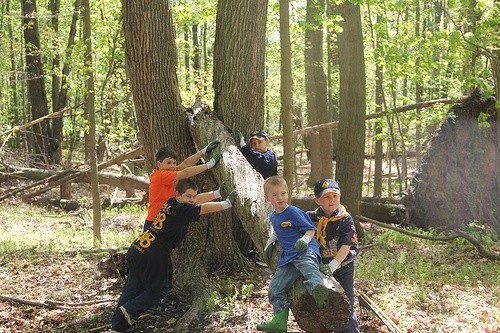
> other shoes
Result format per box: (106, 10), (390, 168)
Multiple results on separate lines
(120, 304), (136, 326)
(111, 327), (138, 333)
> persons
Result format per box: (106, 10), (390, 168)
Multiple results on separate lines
(143, 140), (223, 292)
(256, 176), (329, 333)
(237, 129), (277, 267)
(305, 178), (360, 333)
(110, 178), (238, 333)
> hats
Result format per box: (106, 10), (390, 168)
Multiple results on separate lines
(248, 129), (269, 141)
(314, 178), (341, 199)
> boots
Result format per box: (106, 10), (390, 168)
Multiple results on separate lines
(311, 284), (329, 310)
(256, 309), (289, 332)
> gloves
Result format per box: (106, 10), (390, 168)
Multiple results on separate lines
(205, 150), (222, 170)
(237, 129), (246, 148)
(294, 235), (312, 252)
(263, 234), (278, 264)
(214, 179), (227, 200)
(221, 190), (239, 210)
(201, 139), (220, 156)
(319, 259), (339, 278)
